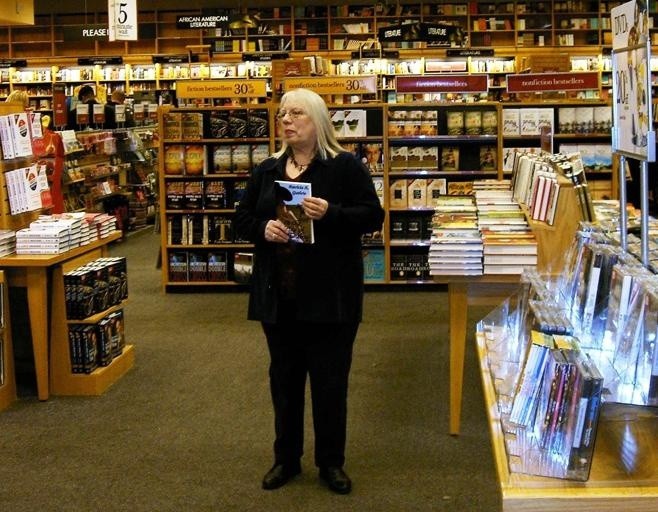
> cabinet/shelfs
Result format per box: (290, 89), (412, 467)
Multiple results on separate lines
(383, 104), (502, 285)
(0, 2), (658, 56)
(499, 104), (615, 197)
(432, 181), (584, 437)
(50, 250), (135, 396)
(161, 54), (658, 102)
(3, 61), (158, 128)
(159, 103), (273, 293)
(273, 103), (386, 286)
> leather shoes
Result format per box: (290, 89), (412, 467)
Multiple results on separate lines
(262, 461), (300, 489)
(319, 467), (353, 493)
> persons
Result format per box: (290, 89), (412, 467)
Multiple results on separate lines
(104, 90), (136, 128)
(228, 86), (386, 494)
(5, 89), (29, 107)
(67, 84), (103, 130)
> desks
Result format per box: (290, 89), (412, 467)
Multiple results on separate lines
(0, 231), (124, 401)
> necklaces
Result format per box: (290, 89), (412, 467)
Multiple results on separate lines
(289, 154), (310, 173)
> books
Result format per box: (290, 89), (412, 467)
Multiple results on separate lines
(257, 23), (290, 34)
(426, 199), (484, 279)
(474, 189), (538, 276)
(51, 130), (77, 152)
(0, 211), (116, 259)
(68, 310), (126, 374)
(507, 266), (604, 479)
(168, 251), (228, 287)
(557, 34), (574, 46)
(330, 108), (368, 137)
(233, 252), (253, 286)
(63, 256), (129, 320)
(4, 166), (54, 215)
(387, 109), (497, 135)
(390, 214), (430, 242)
(390, 254), (432, 281)
(601, 2), (620, 12)
(560, 143), (612, 172)
(168, 180), (246, 209)
(214, 39), (246, 53)
(554, 221), (658, 406)
(165, 109), (268, 138)
(167, 214), (249, 244)
(570, 17), (598, 29)
(517, 19), (526, 30)
(335, 5), (373, 17)
(272, 179), (316, 246)
(361, 247), (385, 280)
(258, 38), (291, 51)
(343, 22), (369, 34)
(163, 144), (269, 174)
(390, 177), (511, 209)
(517, 33), (545, 47)
(388, 145), (498, 170)
(362, 217), (386, 244)
(341, 142), (383, 172)
(0, 113), (44, 159)
(371, 176), (384, 209)
(517, 4), (527, 13)
(554, 0), (585, 12)
(333, 40), (374, 50)
(602, 18), (611, 29)
(503, 105), (613, 134)
(503, 147), (597, 229)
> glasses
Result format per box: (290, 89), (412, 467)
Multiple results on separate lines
(277, 107), (306, 120)
